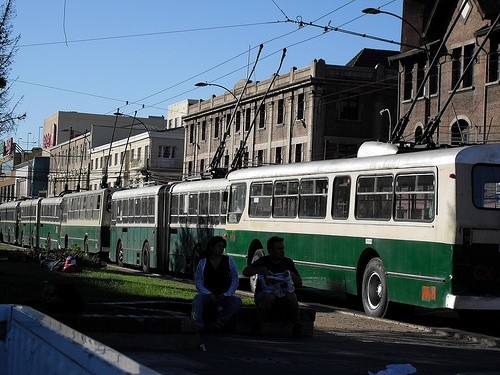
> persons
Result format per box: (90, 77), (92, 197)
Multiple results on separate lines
(242, 236), (307, 338)
(190, 236), (243, 335)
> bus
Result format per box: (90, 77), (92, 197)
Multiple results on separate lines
(1, 1), (500, 321)
